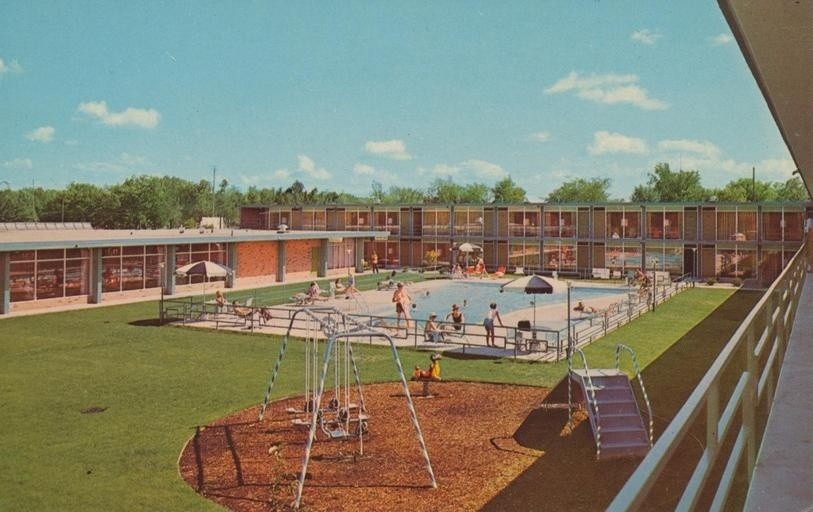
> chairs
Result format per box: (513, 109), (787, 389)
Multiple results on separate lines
(207, 275), (412, 327)
(490, 264), (507, 280)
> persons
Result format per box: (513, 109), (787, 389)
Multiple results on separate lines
(372, 249), (379, 273)
(446, 304), (464, 331)
(452, 254), (484, 274)
(631, 270), (652, 290)
(233, 293), (261, 315)
(803, 207), (813, 273)
(424, 313), (442, 341)
(288, 267), (359, 300)
(392, 281), (412, 339)
(215, 289), (232, 308)
(484, 305), (504, 347)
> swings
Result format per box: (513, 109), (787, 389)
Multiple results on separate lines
(283, 308), (371, 440)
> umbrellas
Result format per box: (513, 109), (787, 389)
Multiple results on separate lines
(175, 260), (232, 303)
(501, 274), (574, 327)
(450, 245), (484, 265)
